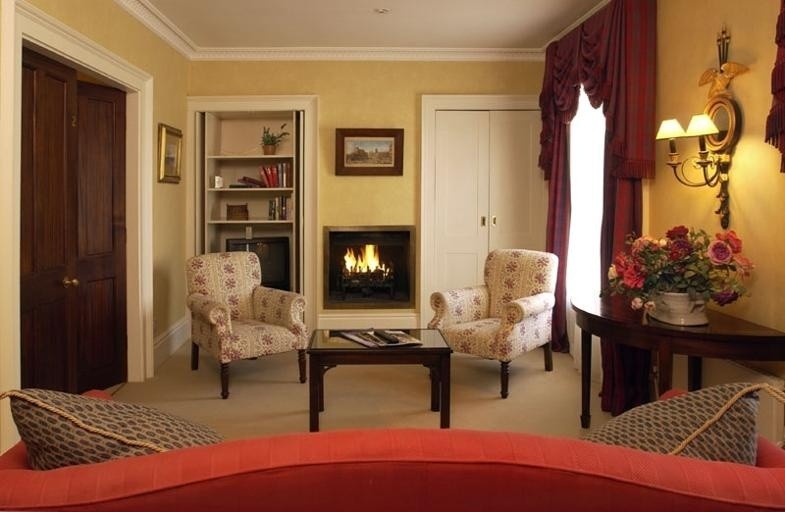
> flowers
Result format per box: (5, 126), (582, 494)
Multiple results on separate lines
(598, 225), (755, 311)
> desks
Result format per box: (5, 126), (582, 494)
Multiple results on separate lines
(570, 294), (785, 429)
(306, 329), (453, 429)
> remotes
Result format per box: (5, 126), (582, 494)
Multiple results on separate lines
(374, 329), (401, 343)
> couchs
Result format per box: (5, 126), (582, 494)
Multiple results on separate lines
(0, 389), (785, 511)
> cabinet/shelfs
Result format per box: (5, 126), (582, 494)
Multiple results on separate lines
(420, 95), (548, 348)
(204, 112), (296, 292)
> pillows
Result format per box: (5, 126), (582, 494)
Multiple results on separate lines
(579, 382), (768, 466)
(0, 388), (228, 471)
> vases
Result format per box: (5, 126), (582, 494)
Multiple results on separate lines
(647, 291), (709, 326)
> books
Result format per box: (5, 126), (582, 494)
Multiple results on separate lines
(229, 161), (293, 221)
(340, 330), (423, 350)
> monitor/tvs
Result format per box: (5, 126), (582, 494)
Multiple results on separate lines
(226, 236), (290, 291)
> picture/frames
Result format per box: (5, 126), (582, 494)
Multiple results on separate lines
(158, 122), (183, 183)
(335, 127), (403, 177)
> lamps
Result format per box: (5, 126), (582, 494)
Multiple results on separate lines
(654, 113), (720, 188)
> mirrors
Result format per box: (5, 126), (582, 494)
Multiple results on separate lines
(701, 94), (738, 153)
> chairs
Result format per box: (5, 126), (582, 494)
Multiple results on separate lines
(426, 249), (559, 398)
(186, 251), (310, 399)
